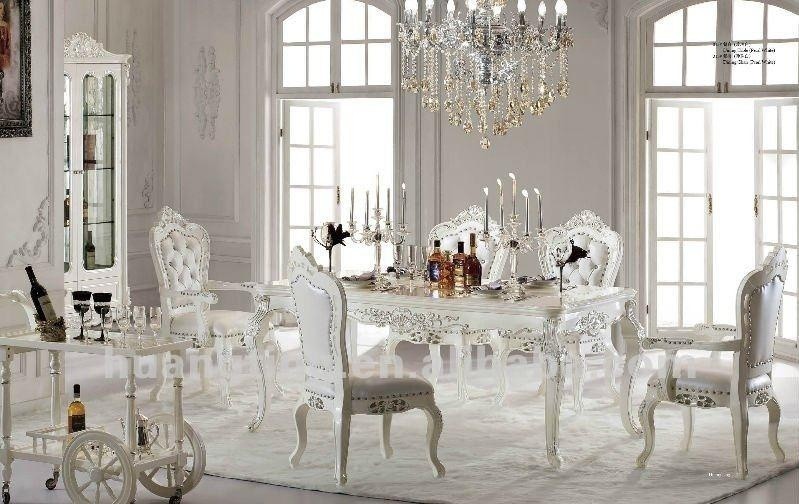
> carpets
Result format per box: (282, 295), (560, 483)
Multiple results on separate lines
(0, 326), (799, 504)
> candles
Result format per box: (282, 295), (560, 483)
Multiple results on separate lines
(509, 173), (516, 215)
(534, 187), (542, 230)
(522, 189), (529, 234)
(376, 172), (379, 209)
(402, 182), (405, 225)
(365, 191), (369, 225)
(483, 185), (489, 231)
(350, 187), (354, 222)
(386, 188), (390, 221)
(497, 178), (503, 228)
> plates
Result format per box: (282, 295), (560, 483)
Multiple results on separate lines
(341, 279), (374, 289)
(522, 281), (558, 291)
(470, 290), (504, 297)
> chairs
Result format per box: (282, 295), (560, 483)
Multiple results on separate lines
(149, 205), (303, 409)
(287, 246), (446, 490)
(411, 205), (510, 402)
(493, 210), (624, 414)
(636, 244), (788, 480)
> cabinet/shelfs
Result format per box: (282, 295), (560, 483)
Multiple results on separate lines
(64, 32), (132, 317)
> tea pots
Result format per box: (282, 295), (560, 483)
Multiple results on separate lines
(118, 408), (159, 460)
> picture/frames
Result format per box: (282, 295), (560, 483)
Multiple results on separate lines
(0, 0), (32, 138)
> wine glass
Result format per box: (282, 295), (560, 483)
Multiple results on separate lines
(79, 306), (92, 346)
(71, 290), (91, 341)
(133, 305), (143, 318)
(149, 305), (161, 339)
(407, 246), (417, 293)
(393, 244), (403, 287)
(94, 293), (112, 343)
(131, 315), (146, 350)
(422, 246), (431, 286)
(115, 306), (131, 349)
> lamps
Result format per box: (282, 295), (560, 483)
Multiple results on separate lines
(396, 0), (576, 150)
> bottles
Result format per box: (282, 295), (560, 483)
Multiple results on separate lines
(83, 191), (88, 223)
(64, 189), (70, 226)
(25, 266), (57, 322)
(452, 242), (468, 287)
(67, 383), (85, 435)
(427, 240), (444, 290)
(438, 251), (455, 297)
(85, 230), (95, 270)
(463, 233), (482, 288)
